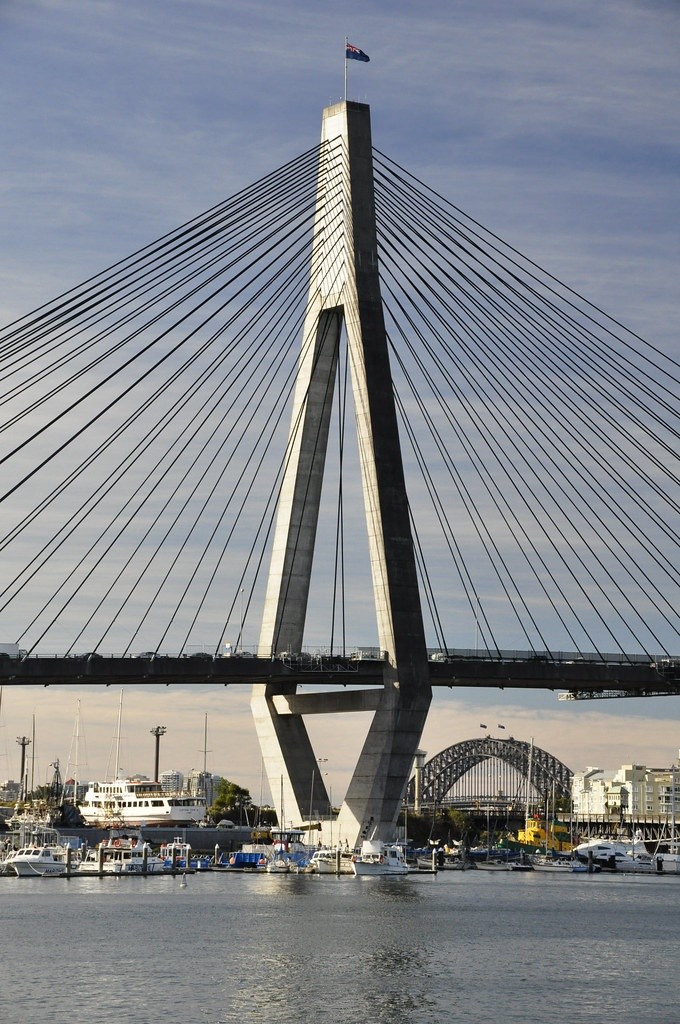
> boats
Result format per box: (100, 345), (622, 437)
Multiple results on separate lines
(77, 778), (206, 828)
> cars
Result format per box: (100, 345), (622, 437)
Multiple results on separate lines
(76, 652), (103, 659)
(132, 652), (160, 658)
(186, 652), (213, 659)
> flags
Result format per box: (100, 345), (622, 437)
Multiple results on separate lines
(498, 724), (505, 729)
(346, 43), (370, 62)
(480, 723), (487, 729)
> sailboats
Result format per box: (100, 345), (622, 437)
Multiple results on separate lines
(0, 690), (680, 879)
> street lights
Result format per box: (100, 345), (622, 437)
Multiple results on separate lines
(150, 725), (167, 782)
(16, 736), (32, 800)
(240, 588), (244, 650)
(476, 597), (481, 657)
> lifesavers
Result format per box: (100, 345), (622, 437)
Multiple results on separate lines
(114, 839), (122, 847)
(130, 839), (138, 847)
(380, 856), (383, 864)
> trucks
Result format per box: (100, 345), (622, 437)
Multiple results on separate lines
(0, 642), (30, 658)
